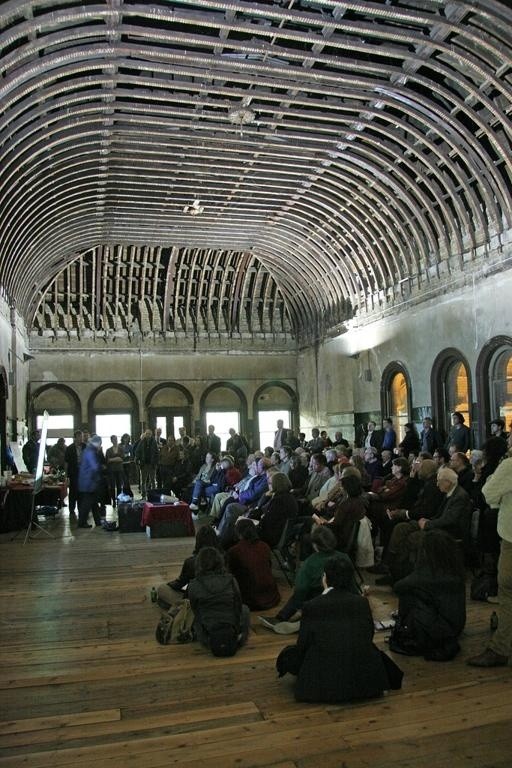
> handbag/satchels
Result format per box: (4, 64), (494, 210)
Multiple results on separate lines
(210, 622), (239, 657)
(470, 570), (498, 601)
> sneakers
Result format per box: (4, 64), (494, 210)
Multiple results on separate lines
(486, 595), (499, 604)
(366, 563), (390, 574)
(375, 575), (394, 585)
(467, 648), (508, 667)
(256, 615), (282, 630)
(189, 502), (199, 510)
(78, 522), (92, 528)
(191, 512), (199, 519)
(273, 618), (301, 635)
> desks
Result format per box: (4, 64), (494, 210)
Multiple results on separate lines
(117, 500), (147, 532)
(143, 500), (196, 539)
(0, 479), (72, 533)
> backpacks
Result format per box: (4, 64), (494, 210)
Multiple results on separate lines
(155, 599), (196, 645)
(383, 605), (460, 661)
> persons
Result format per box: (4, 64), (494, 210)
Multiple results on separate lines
(21, 429), (43, 477)
(258, 522), (361, 634)
(187, 543), (251, 649)
(389, 529), (467, 662)
(49, 412), (511, 609)
(467, 455), (512, 667)
(275, 553), (405, 704)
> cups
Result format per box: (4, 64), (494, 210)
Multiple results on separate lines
(4, 471), (13, 480)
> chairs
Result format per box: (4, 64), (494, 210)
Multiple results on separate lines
(263, 507), (498, 592)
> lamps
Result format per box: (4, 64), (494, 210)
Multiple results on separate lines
(183, 201), (204, 217)
(12, 408), (61, 547)
(225, 104), (258, 126)
